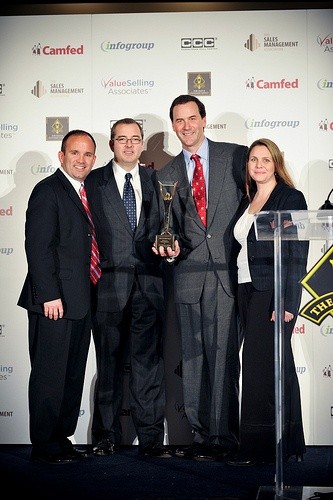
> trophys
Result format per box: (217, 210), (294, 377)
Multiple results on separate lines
(154, 179), (181, 251)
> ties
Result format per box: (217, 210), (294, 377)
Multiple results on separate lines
(191, 154), (207, 230)
(122, 174), (137, 236)
(79, 183), (102, 285)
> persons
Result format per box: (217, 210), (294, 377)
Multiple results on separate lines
(83, 119), (169, 459)
(15, 96), (98, 461)
(150, 95), (251, 462)
(230, 139), (311, 464)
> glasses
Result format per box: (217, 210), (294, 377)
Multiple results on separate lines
(115, 137), (142, 144)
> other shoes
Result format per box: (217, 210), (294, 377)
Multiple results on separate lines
(30, 442), (88, 464)
(188, 446), (214, 462)
(168, 446), (189, 458)
(92, 441), (119, 456)
(150, 446), (174, 458)
